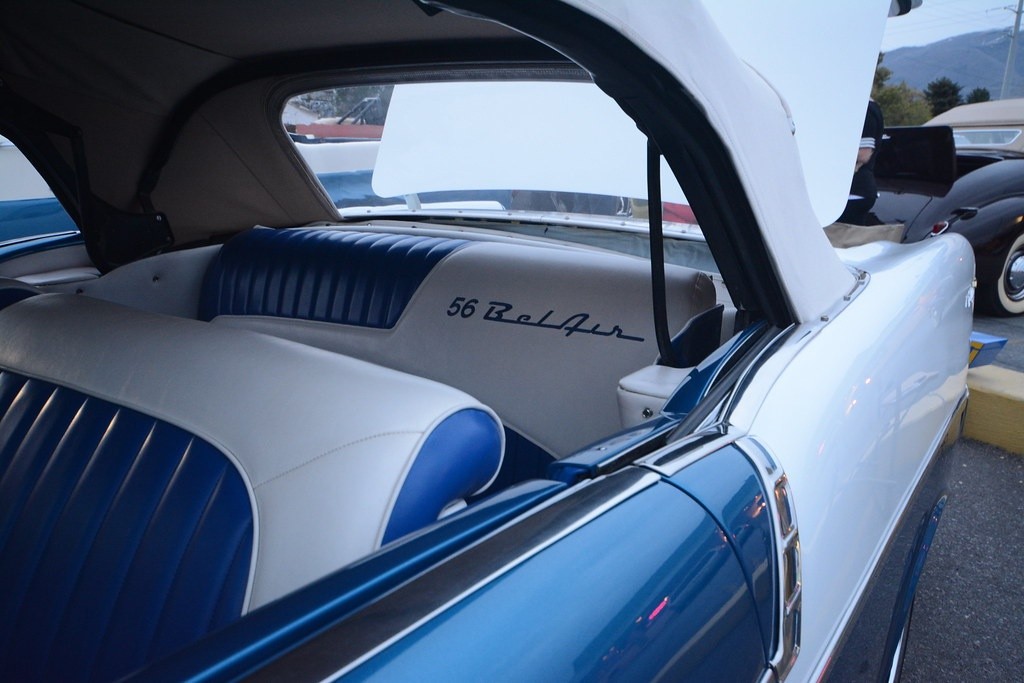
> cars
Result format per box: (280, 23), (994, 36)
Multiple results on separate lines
(0, 0), (978, 683)
(836, 97), (1024, 317)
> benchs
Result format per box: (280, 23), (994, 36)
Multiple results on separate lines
(195, 225), (715, 505)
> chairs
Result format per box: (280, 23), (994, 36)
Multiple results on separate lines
(0, 292), (506, 683)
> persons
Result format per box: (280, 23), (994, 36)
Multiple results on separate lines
(837, 98), (884, 225)
